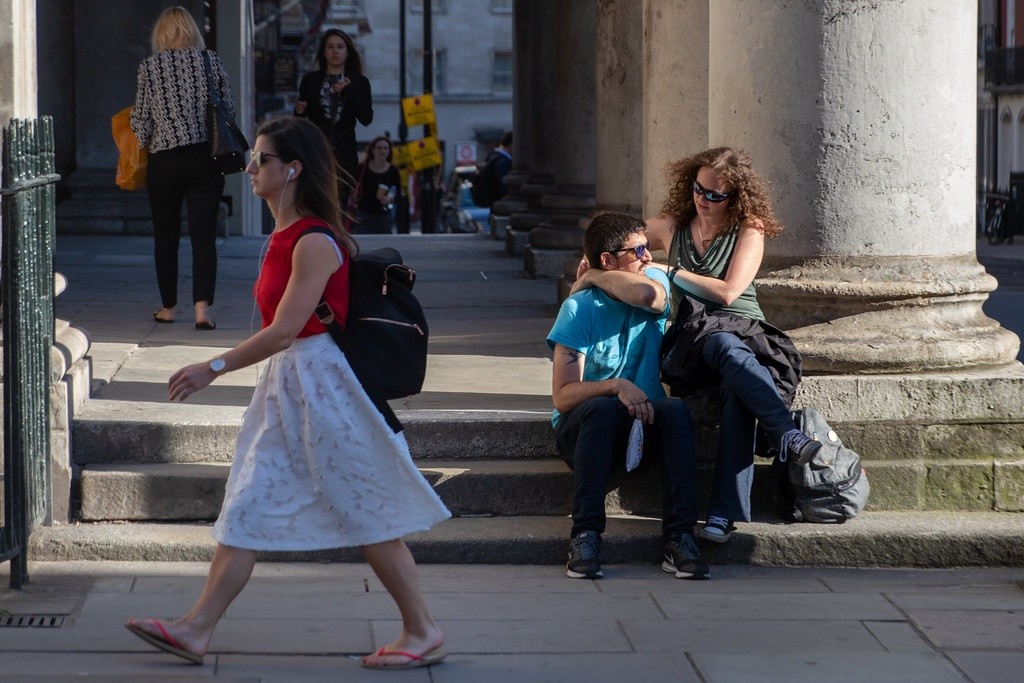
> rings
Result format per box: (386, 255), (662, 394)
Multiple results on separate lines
(644, 398), (652, 404)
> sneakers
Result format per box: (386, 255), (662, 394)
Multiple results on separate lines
(566, 529), (603, 578)
(662, 534), (710, 579)
(700, 515), (734, 542)
(779, 429), (823, 466)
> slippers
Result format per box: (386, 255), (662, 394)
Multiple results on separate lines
(362, 645), (448, 669)
(195, 319), (216, 330)
(123, 619), (203, 664)
(153, 309), (174, 324)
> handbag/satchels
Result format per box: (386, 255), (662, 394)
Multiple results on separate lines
(111, 106), (150, 191)
(208, 96), (250, 175)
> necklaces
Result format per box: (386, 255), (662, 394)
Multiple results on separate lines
(697, 217), (724, 253)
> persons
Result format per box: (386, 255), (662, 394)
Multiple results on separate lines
(121, 117), (452, 670)
(546, 211), (712, 580)
(575, 145), (822, 543)
(293, 29), (373, 233)
(484, 130), (513, 225)
(358, 136), (401, 235)
(128, 5), (237, 331)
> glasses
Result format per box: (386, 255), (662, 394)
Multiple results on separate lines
(612, 241), (651, 259)
(248, 149), (281, 167)
(691, 180), (732, 203)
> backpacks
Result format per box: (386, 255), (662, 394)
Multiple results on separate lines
(774, 406), (871, 524)
(294, 227), (429, 434)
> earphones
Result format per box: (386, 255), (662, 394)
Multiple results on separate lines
(286, 168), (295, 181)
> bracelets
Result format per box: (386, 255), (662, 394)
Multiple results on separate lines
(666, 265), (676, 277)
(669, 265), (686, 282)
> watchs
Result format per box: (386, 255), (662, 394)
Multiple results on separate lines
(208, 354), (226, 377)
